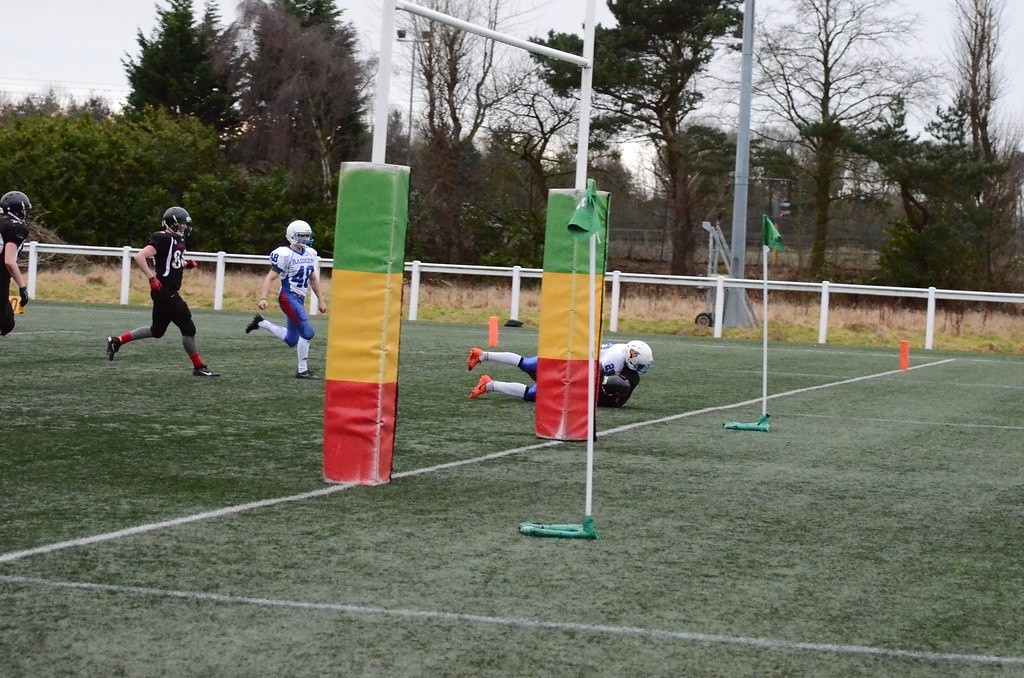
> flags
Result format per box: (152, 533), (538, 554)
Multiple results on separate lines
(762, 214), (784, 253)
(567, 177), (601, 242)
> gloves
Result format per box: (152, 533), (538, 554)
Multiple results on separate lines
(185, 259), (197, 269)
(19, 286), (28, 307)
(149, 277), (162, 293)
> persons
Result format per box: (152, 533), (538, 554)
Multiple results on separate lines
(0, 191), (33, 339)
(597, 368), (640, 408)
(105, 206), (221, 377)
(243, 220), (327, 380)
(466, 339), (653, 403)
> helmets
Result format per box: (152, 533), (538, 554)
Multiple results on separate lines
(0, 191), (32, 222)
(286, 220), (314, 248)
(624, 340), (653, 375)
(162, 207), (193, 243)
(600, 374), (630, 401)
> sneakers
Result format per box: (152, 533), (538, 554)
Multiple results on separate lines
(468, 347), (482, 371)
(106, 337), (119, 361)
(246, 314), (264, 333)
(193, 365), (220, 377)
(470, 375), (491, 399)
(296, 370), (320, 380)
